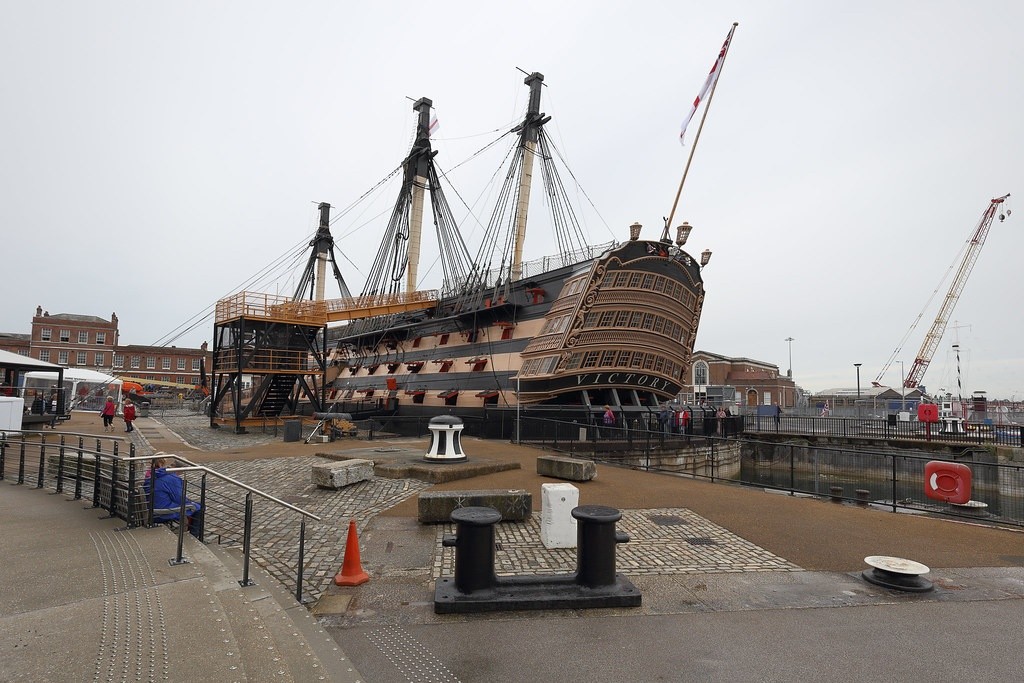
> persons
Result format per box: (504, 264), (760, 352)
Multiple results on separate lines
(144, 451), (204, 538)
(603, 401), (783, 437)
(1, 382), (155, 432)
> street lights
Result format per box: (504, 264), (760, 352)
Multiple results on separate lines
(895, 359), (906, 410)
(785, 337), (795, 380)
(853, 363), (863, 419)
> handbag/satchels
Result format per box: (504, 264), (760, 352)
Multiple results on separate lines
(100, 413), (104, 418)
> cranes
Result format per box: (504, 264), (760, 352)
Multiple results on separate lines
(871, 190), (1011, 388)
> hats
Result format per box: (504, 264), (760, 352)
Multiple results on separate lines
(603, 405), (610, 408)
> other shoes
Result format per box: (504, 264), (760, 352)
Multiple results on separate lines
(130, 428), (133, 431)
(52, 427), (56, 429)
(125, 430), (129, 432)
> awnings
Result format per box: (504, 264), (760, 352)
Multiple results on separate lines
(323, 287), (543, 398)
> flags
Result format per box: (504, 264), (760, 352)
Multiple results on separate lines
(676, 27), (732, 146)
(821, 401), (828, 418)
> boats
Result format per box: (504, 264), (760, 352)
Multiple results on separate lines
(285, 17), (741, 435)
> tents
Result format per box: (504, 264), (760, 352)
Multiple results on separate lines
(0, 349), (124, 413)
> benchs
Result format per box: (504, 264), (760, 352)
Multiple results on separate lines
(333, 420), (374, 440)
(99, 471), (196, 534)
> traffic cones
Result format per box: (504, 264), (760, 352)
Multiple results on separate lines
(335, 519), (370, 587)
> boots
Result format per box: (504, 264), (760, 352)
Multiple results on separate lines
(110, 424), (115, 431)
(104, 427), (109, 432)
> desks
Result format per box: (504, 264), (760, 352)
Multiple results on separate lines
(314, 412), (352, 442)
